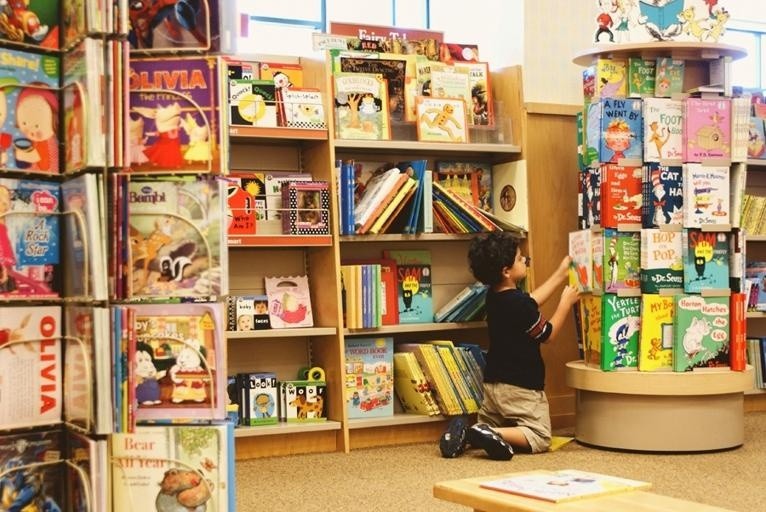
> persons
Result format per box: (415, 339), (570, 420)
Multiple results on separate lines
(440, 231), (581, 461)
(595, 7), (615, 43)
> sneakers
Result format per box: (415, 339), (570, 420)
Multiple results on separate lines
(470, 423), (514, 460)
(440, 418), (467, 458)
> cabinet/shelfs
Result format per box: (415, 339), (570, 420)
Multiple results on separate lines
(220, 54), (349, 462)
(743, 154), (766, 411)
(326, 55), (533, 453)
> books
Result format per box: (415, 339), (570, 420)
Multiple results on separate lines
(639, 0), (685, 32)
(478, 468), (653, 505)
